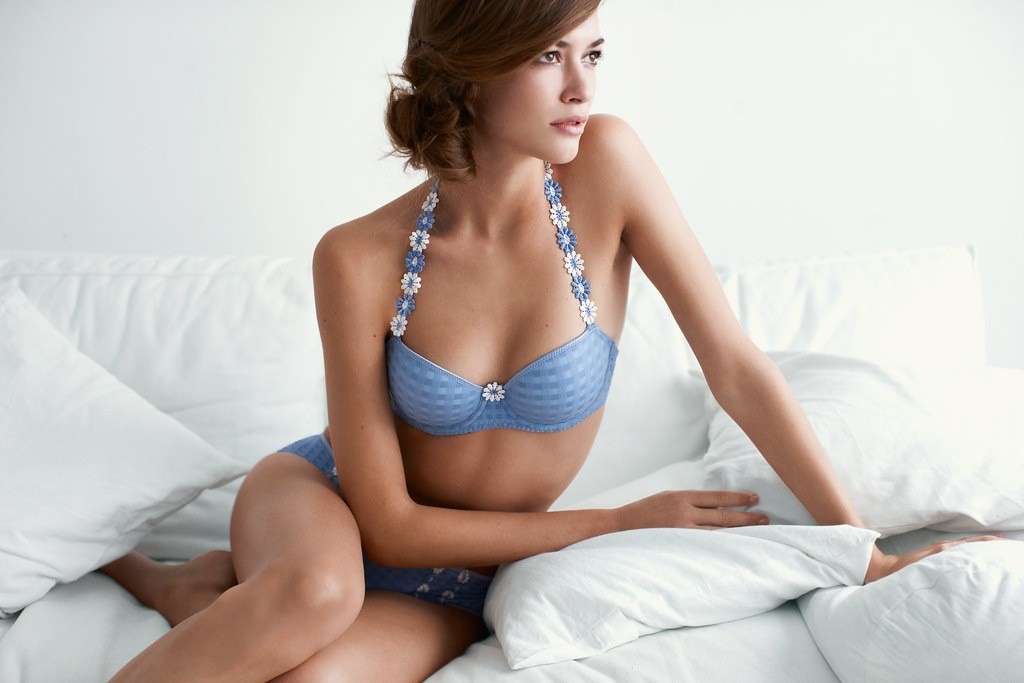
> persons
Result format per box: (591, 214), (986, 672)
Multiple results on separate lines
(98, 0), (1007, 683)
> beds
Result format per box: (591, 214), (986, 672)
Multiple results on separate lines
(0, 244), (1024, 683)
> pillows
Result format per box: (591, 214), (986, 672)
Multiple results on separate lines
(0, 274), (244, 620)
(478, 348), (1024, 683)
